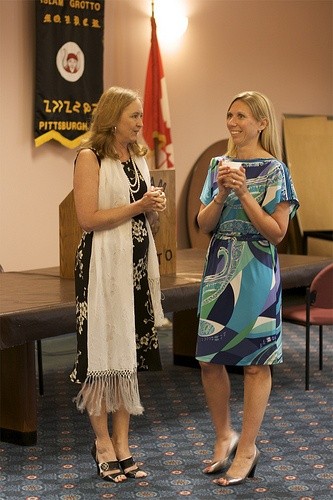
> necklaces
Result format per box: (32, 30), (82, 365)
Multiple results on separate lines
(124, 155), (140, 203)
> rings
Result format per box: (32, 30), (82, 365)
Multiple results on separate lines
(233, 180), (237, 184)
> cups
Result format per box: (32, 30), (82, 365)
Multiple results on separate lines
(222, 161), (243, 170)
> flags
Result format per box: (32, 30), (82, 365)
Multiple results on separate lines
(142, 17), (175, 171)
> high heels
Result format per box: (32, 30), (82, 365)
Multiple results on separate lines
(204, 430), (239, 474)
(91, 439), (128, 483)
(217, 447), (260, 486)
(110, 435), (147, 479)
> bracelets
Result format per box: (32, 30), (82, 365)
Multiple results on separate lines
(214, 196), (224, 205)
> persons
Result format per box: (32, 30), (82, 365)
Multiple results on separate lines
(68, 87), (167, 484)
(195, 91), (300, 486)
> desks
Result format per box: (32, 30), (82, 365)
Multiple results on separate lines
(0, 248), (333, 447)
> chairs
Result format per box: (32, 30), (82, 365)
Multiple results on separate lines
(281, 264), (333, 390)
